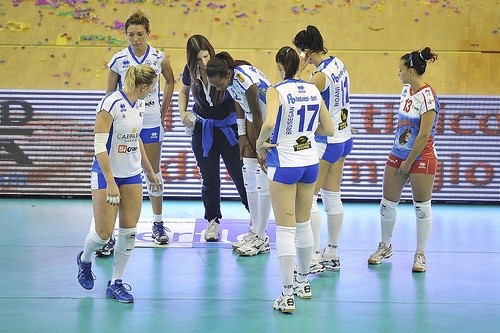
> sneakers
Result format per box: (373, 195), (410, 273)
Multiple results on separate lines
(272, 292), (296, 313)
(236, 234), (270, 256)
(151, 221), (171, 244)
(367, 242), (393, 264)
(232, 229), (256, 251)
(412, 250), (427, 271)
(292, 275), (312, 300)
(320, 254), (341, 272)
(105, 279), (134, 303)
(96, 237), (116, 256)
(294, 259), (327, 274)
(76, 251), (97, 290)
(204, 217), (220, 241)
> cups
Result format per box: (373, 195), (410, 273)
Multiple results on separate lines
(186, 113), (196, 135)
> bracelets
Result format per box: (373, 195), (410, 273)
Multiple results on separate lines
(298, 68), (302, 73)
(236, 119), (246, 136)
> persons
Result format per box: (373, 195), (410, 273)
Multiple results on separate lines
(177, 25), (353, 313)
(368, 47), (439, 273)
(77, 65), (163, 303)
(94, 9), (174, 257)
